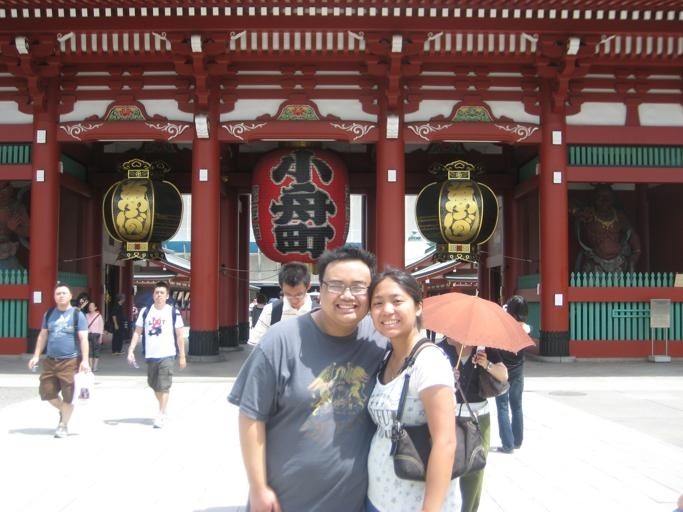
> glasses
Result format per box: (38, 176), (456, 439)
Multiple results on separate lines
(323, 281), (369, 295)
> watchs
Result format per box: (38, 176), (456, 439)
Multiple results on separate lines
(487, 362), (493, 371)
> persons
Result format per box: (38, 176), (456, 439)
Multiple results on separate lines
(250, 294), (278, 329)
(311, 296), (320, 307)
(437, 336), (507, 512)
(126, 280), (187, 428)
(426, 329), (436, 342)
(230, 250), (389, 511)
(28, 283), (89, 437)
(485, 295), (531, 453)
(366, 270), (463, 512)
(85, 303), (104, 371)
(109, 293), (126, 356)
(248, 263), (320, 347)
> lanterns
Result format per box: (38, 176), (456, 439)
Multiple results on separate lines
(414, 160), (499, 261)
(100, 157), (184, 264)
(250, 144), (349, 275)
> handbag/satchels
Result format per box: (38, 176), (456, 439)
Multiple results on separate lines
(392, 418), (486, 481)
(72, 372), (95, 406)
(477, 370), (510, 399)
